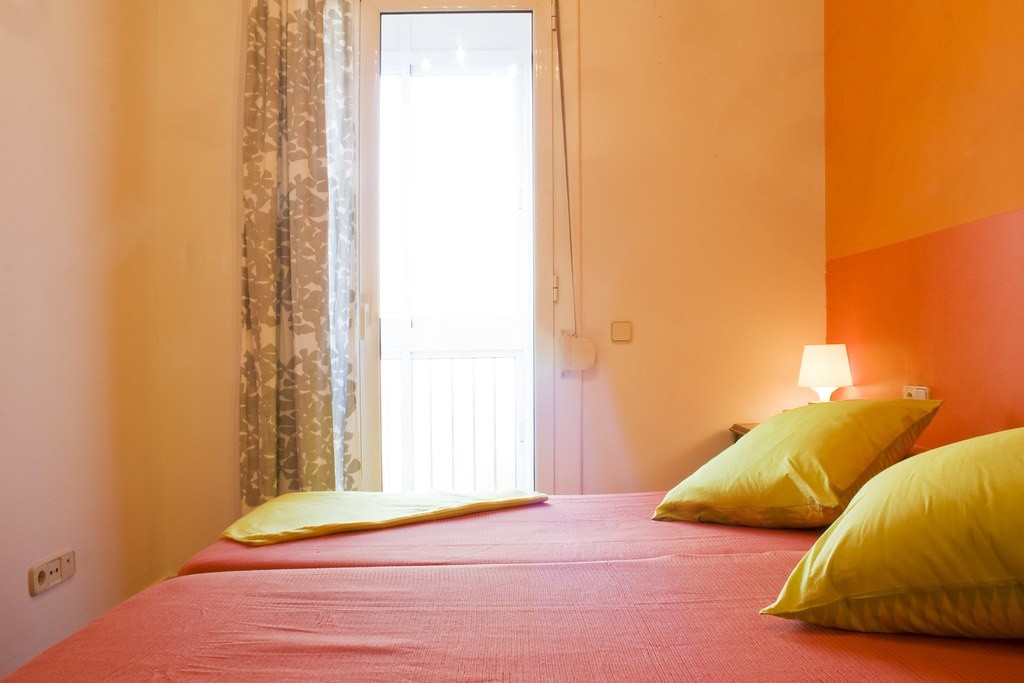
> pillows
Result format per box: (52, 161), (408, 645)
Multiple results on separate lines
(651, 398), (944, 527)
(758, 426), (1024, 637)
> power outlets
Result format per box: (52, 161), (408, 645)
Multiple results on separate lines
(28, 548), (76, 596)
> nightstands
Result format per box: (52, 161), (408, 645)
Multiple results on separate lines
(729, 423), (759, 442)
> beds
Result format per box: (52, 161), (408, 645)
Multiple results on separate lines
(0, 490), (1024, 683)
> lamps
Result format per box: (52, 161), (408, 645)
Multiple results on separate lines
(796, 343), (854, 402)
